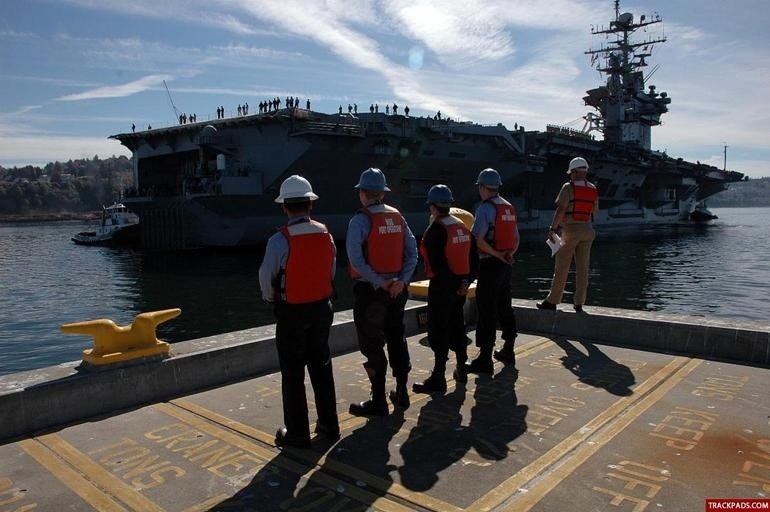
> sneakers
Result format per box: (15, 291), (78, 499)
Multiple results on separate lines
(536, 297), (559, 316)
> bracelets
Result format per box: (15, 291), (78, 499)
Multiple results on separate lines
(547, 225), (557, 232)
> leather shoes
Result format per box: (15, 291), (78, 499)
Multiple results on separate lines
(273, 423), (311, 449)
(312, 416), (342, 438)
(573, 303), (584, 311)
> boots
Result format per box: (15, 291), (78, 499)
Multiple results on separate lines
(452, 342), (473, 385)
(390, 364), (415, 412)
(492, 328), (521, 369)
(466, 336), (499, 377)
(349, 362), (391, 418)
(413, 347), (450, 395)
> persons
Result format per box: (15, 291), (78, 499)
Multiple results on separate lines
(412, 184), (479, 394)
(132, 123), (135, 132)
(369, 104), (374, 113)
(237, 104), (242, 117)
(348, 104), (352, 112)
(375, 104), (378, 111)
(307, 99), (311, 110)
(437, 110), (441, 120)
(353, 103), (358, 113)
(257, 175), (343, 448)
(404, 105), (410, 116)
(189, 113), (193, 122)
(537, 156), (600, 312)
(220, 106), (224, 118)
(179, 113), (183, 125)
(393, 103), (399, 115)
(193, 113), (197, 122)
(346, 167), (411, 416)
(545, 123), (596, 141)
(339, 105), (343, 114)
(183, 113), (187, 124)
(217, 107), (221, 119)
(242, 105), (245, 115)
(386, 105), (389, 114)
(465, 168), (519, 372)
(257, 96), (300, 114)
(245, 102), (249, 114)
(148, 123), (152, 129)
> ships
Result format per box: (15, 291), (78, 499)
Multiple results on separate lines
(106, 1), (748, 252)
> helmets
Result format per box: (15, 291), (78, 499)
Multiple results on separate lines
(565, 154), (592, 175)
(353, 166), (396, 194)
(424, 181), (458, 208)
(475, 165), (508, 190)
(270, 173), (322, 205)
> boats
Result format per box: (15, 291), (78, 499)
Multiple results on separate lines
(72, 192), (138, 246)
